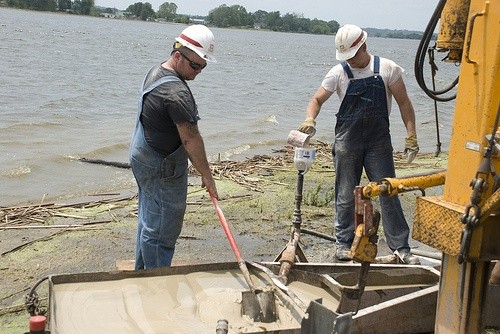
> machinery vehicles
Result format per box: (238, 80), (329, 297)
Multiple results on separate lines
(18, 0), (500, 334)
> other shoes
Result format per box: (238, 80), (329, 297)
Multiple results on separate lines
(335, 246), (353, 261)
(400, 252), (421, 265)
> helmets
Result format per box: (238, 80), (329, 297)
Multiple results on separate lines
(334, 24), (367, 60)
(174, 24), (217, 64)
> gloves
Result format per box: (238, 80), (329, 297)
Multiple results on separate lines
(298, 119), (316, 137)
(403, 134), (419, 164)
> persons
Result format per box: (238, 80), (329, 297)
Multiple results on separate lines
(298, 24), (419, 265)
(129, 25), (217, 270)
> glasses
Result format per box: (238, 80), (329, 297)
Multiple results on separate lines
(170, 49), (207, 70)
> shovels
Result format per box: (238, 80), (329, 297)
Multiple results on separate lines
(204, 186), (278, 323)
